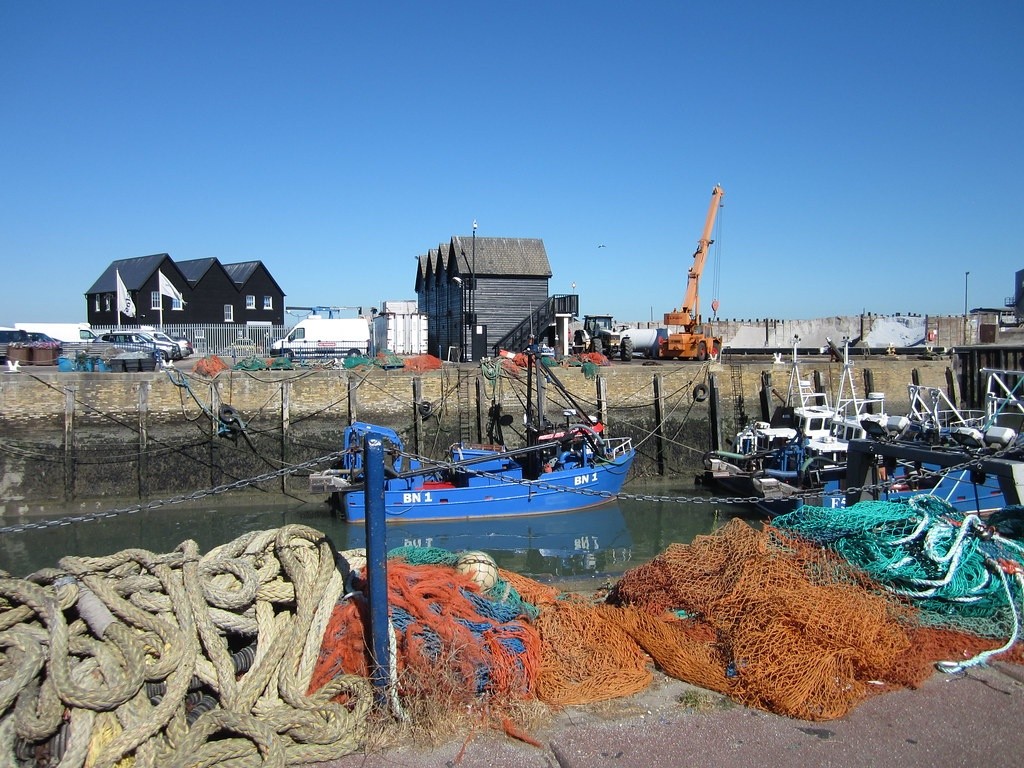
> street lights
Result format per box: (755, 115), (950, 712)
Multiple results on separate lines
(963, 271), (970, 345)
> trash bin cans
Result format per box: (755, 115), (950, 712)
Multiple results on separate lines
(980, 324), (998, 344)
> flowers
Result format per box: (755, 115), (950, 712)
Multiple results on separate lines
(9, 340), (61, 349)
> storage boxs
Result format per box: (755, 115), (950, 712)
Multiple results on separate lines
(451, 445), (523, 487)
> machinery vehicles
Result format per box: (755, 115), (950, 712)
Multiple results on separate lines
(572, 314), (633, 363)
(652, 181), (726, 361)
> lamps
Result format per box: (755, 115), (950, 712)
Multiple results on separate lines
(860, 416), (911, 443)
(950, 426), (1016, 452)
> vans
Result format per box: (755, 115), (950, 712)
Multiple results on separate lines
(270, 315), (370, 361)
(13, 321), (99, 343)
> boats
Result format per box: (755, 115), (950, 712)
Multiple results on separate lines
(702, 332), (1024, 522)
(308, 301), (636, 525)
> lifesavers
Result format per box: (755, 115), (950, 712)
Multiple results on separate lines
(694, 383), (710, 400)
(218, 404), (238, 423)
(418, 402), (431, 414)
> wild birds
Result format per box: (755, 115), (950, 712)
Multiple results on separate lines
(598, 245), (606, 249)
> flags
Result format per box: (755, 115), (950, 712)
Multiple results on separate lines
(118, 274), (136, 318)
(160, 272), (187, 307)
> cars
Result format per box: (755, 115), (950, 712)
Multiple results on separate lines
(231, 338), (255, 355)
(0, 326), (64, 365)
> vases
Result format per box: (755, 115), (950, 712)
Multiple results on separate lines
(6, 345), (59, 366)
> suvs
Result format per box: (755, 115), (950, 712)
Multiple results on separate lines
(92, 332), (195, 363)
